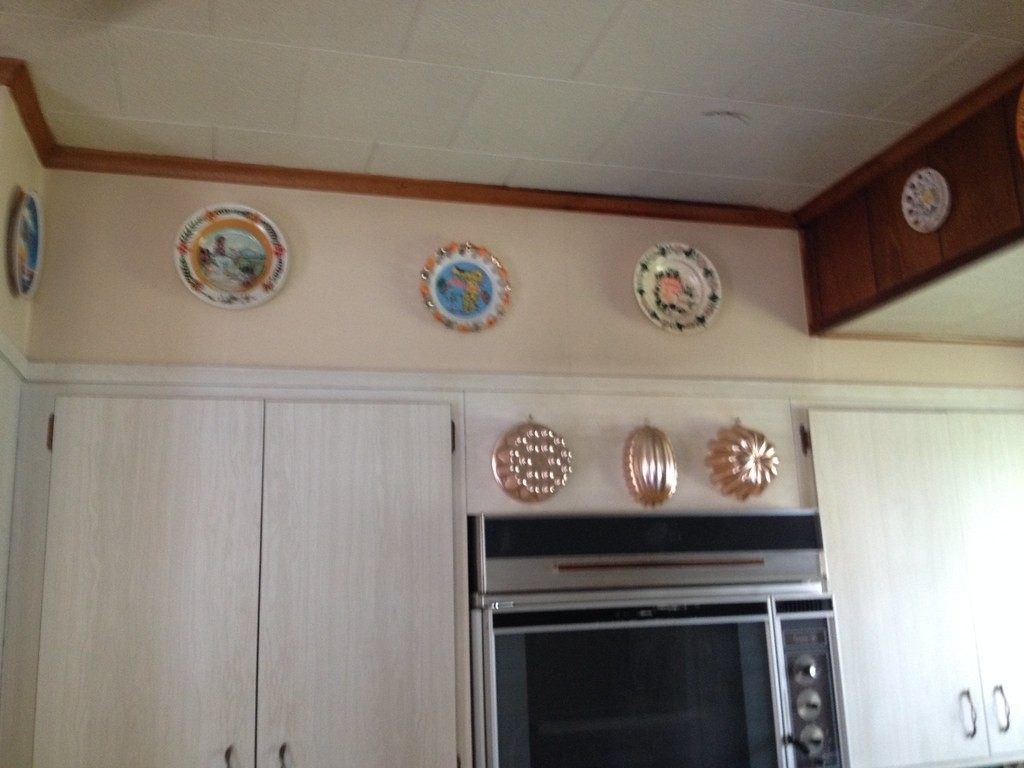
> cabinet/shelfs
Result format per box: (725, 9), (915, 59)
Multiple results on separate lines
(807, 383), (1024, 768)
(14, 363), (471, 768)
